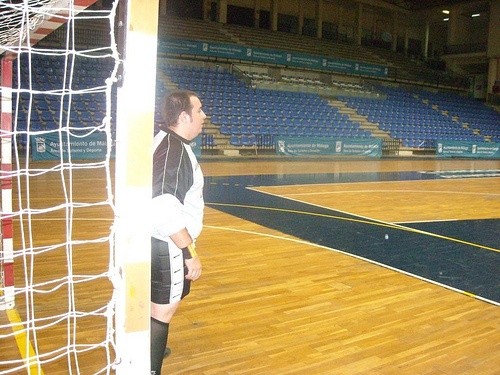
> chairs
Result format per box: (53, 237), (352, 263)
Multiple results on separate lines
(11, 65), (499, 151)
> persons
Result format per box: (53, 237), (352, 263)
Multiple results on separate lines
(152, 89), (206, 375)
